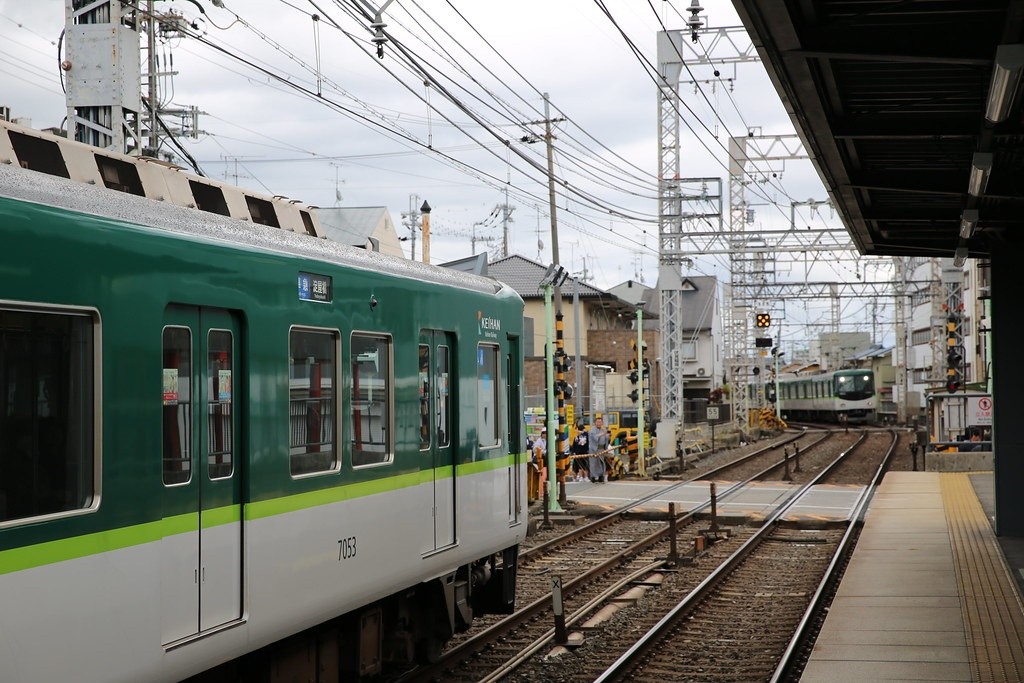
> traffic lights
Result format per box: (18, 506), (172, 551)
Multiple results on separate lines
(756, 314), (771, 327)
(625, 372), (639, 403)
(946, 352), (962, 394)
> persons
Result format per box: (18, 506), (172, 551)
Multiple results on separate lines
(963, 429), (980, 443)
(589, 417), (608, 483)
(532, 428), (547, 466)
(570, 425), (590, 482)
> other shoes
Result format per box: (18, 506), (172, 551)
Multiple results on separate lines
(573, 478), (577, 482)
(597, 476), (604, 483)
(584, 477), (591, 483)
(590, 477), (596, 483)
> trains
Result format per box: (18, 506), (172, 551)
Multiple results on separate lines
(738, 368), (880, 427)
(0, 117), (528, 683)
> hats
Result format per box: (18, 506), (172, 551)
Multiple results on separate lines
(540, 427), (547, 431)
